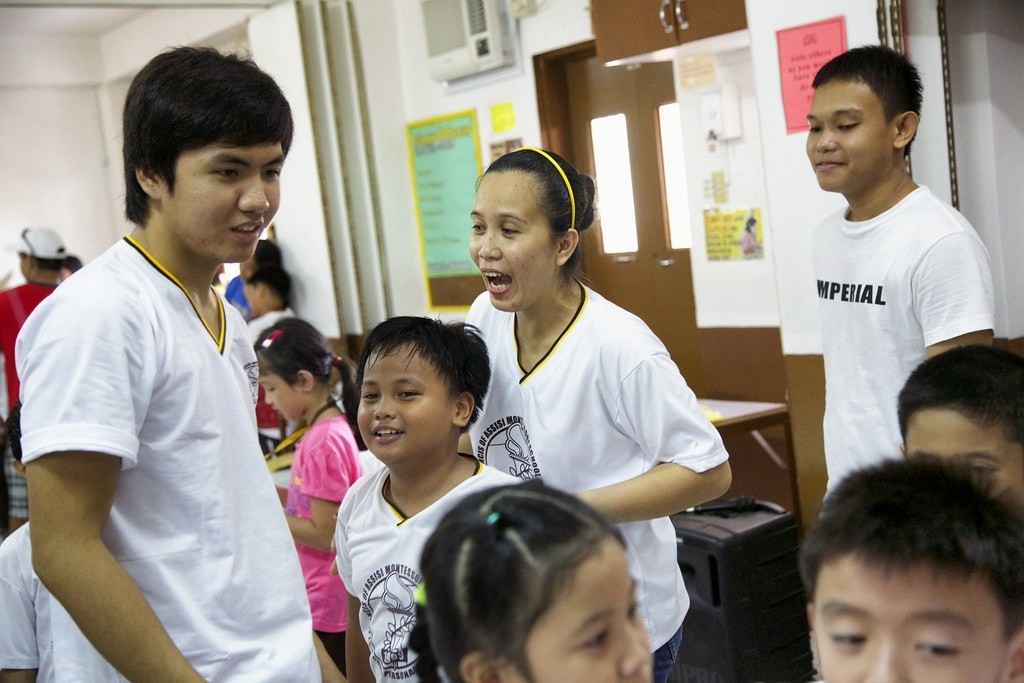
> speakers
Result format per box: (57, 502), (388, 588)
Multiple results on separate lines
(668, 498), (818, 683)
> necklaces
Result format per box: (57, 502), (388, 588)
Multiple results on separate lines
(307, 397), (338, 429)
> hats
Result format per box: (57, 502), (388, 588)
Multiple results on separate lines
(17, 227), (66, 259)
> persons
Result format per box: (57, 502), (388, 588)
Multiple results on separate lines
(332, 315), (524, 683)
(406, 477), (664, 683)
(804, 43), (998, 512)
(12, 42), (321, 683)
(222, 242), (361, 676)
(0, 401), (41, 683)
(798, 453), (1024, 682)
(896, 344), (1023, 503)
(2, 224), (86, 535)
(457, 146), (733, 683)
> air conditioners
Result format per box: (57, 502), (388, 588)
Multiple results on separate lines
(418, 0), (514, 82)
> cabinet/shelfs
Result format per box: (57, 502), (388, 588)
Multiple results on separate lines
(589, 0), (752, 66)
